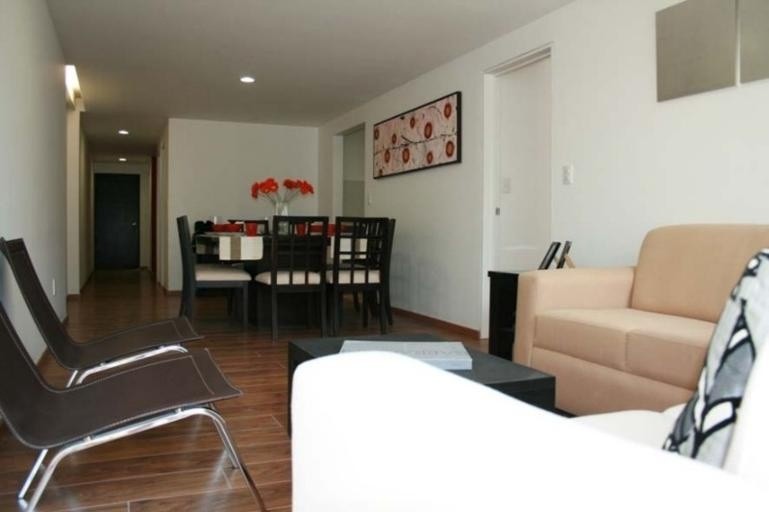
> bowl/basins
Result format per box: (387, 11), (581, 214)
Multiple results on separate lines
(210, 222), (242, 232)
(310, 224), (348, 234)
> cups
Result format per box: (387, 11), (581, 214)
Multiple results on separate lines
(297, 223), (306, 235)
(246, 221), (258, 236)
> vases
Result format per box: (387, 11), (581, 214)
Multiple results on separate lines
(274, 201), (289, 235)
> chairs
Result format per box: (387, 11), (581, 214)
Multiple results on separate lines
(176, 215), (397, 342)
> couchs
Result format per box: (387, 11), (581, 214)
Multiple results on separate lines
(511, 222), (769, 418)
(289, 346), (768, 512)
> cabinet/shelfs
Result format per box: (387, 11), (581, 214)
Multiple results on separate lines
(487, 269), (517, 359)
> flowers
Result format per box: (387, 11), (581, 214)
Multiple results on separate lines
(250, 176), (314, 217)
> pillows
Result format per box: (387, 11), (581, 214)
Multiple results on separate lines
(664, 249), (768, 469)
